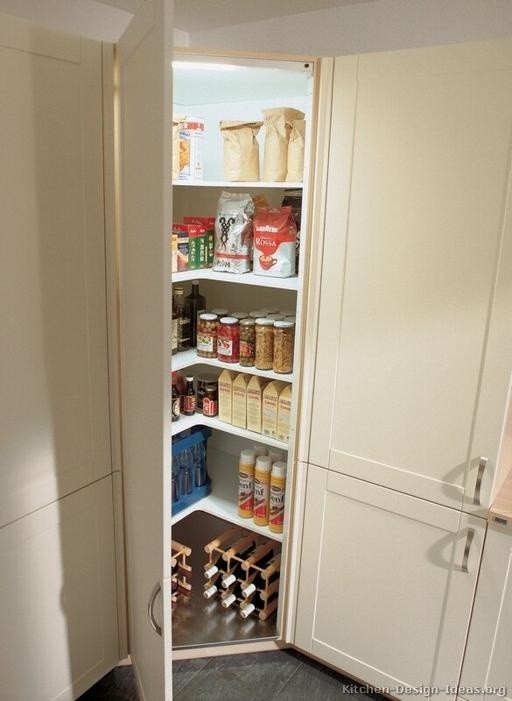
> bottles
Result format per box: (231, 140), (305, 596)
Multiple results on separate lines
(183, 374), (197, 416)
(204, 533), (280, 617)
(172, 426), (210, 503)
(219, 369), (293, 443)
(171, 302), (177, 353)
(175, 286), (193, 353)
(237, 443), (287, 533)
(170, 370), (181, 423)
(187, 280), (207, 347)
(201, 384), (218, 417)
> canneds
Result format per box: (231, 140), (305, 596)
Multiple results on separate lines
(238, 318), (256, 368)
(195, 313), (221, 360)
(254, 318), (275, 371)
(272, 320), (294, 374)
(216, 316), (240, 364)
(195, 373), (219, 409)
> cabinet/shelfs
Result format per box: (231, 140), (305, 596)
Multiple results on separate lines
(114, 2), (320, 701)
(0, 471), (129, 701)
(306, 38), (511, 521)
(283, 460), (488, 701)
(2, 14), (120, 532)
